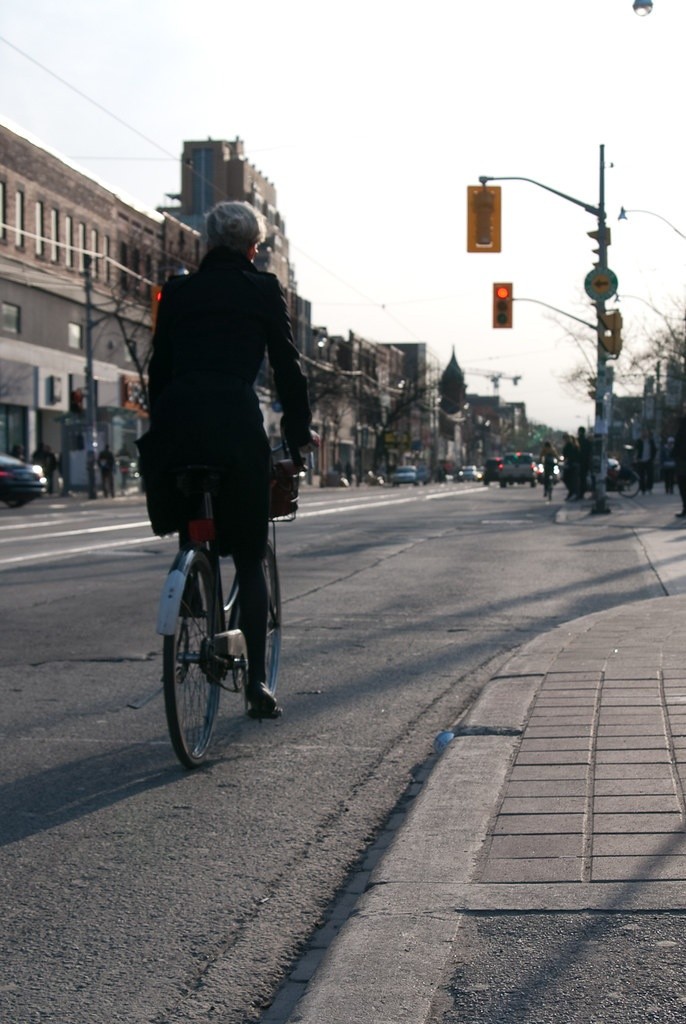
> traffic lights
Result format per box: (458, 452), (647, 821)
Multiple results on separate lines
(587, 228), (610, 268)
(493, 282), (513, 329)
(151, 286), (164, 331)
(598, 309), (623, 356)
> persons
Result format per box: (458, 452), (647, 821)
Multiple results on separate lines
(11, 441), (134, 500)
(538, 427), (593, 503)
(632, 414), (686, 518)
(134, 199), (314, 712)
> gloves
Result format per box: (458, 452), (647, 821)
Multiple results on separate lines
(302, 430), (320, 452)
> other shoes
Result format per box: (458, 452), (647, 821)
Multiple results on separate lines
(180, 570), (204, 619)
(249, 680), (277, 725)
(674, 511), (685, 516)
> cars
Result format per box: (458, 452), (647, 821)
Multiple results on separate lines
(459, 465), (482, 482)
(319, 471), (351, 488)
(0, 453), (49, 508)
(483, 458), (503, 484)
(499, 453), (536, 488)
(393, 465), (426, 485)
(538, 466), (561, 484)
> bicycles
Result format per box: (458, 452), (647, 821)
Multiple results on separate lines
(538, 461), (558, 500)
(609, 464), (641, 497)
(156, 443), (308, 768)
